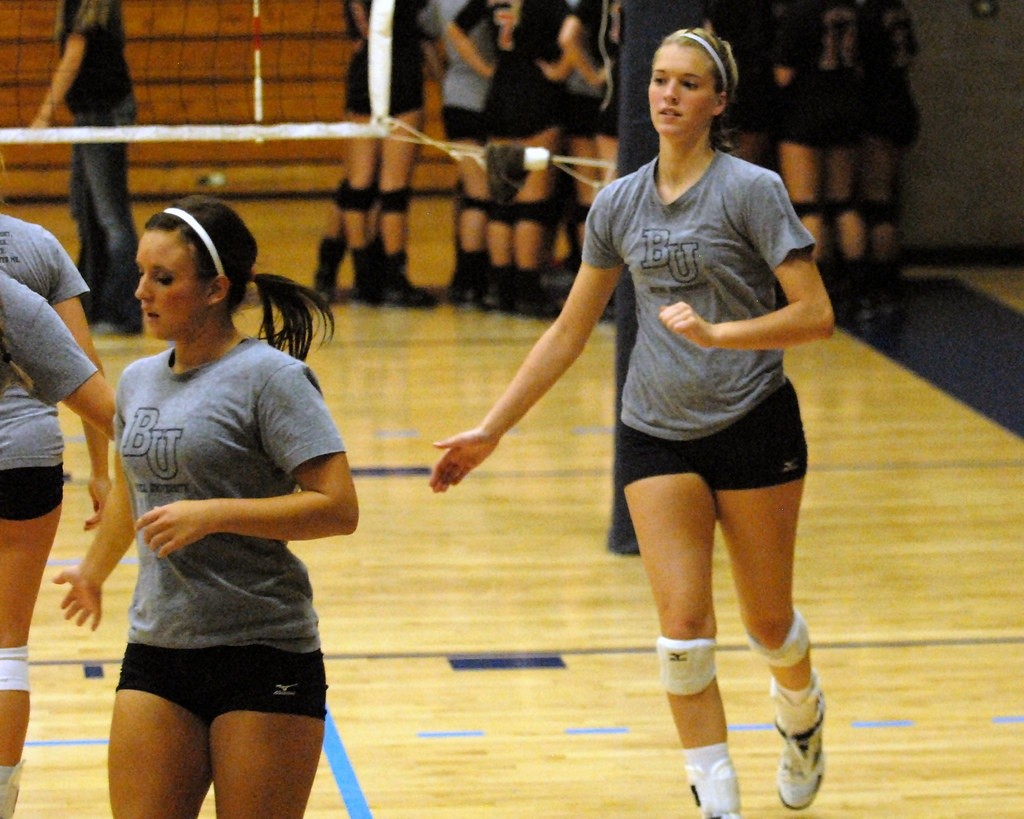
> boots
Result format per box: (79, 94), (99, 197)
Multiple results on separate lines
(684, 758), (741, 819)
(314, 245), (569, 320)
(774, 691), (825, 810)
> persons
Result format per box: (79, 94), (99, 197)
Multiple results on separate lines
(26, 0), (141, 335)
(429, 27), (836, 819)
(50, 199), (360, 819)
(0, 211), (118, 819)
(314, 0), (920, 313)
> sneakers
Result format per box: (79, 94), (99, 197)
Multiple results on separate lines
(1, 781), (20, 816)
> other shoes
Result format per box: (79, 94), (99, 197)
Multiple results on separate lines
(90, 318), (145, 337)
(826, 262), (907, 306)
